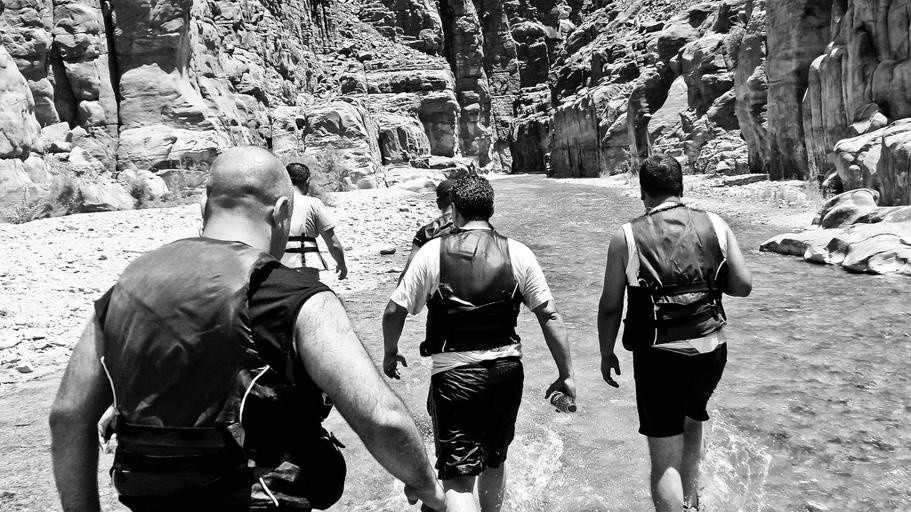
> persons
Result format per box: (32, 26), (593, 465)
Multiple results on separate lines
(45, 142), (452, 511)
(592, 152), (758, 512)
(377, 168), (579, 510)
(394, 179), (454, 289)
(274, 160), (351, 287)
